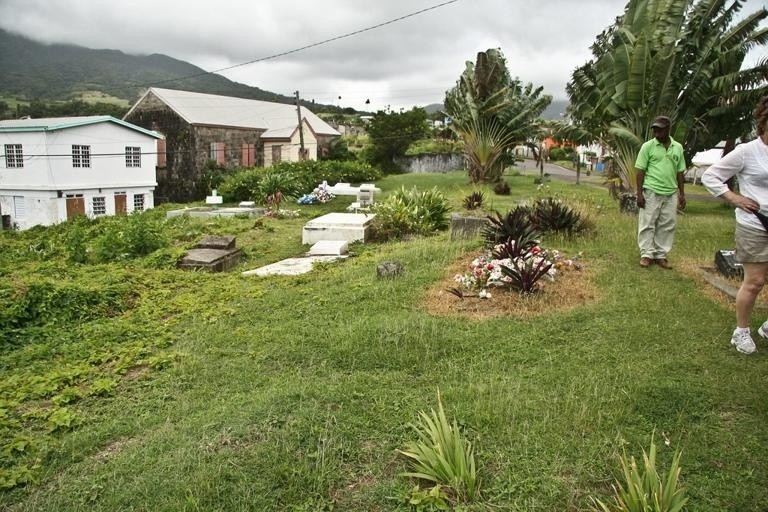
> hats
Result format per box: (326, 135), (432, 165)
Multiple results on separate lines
(650, 115), (671, 129)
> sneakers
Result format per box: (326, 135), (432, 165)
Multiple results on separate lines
(757, 319), (768, 341)
(730, 328), (758, 356)
(654, 257), (672, 270)
(639, 257), (653, 267)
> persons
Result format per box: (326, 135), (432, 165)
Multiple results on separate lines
(700, 96), (768, 355)
(635, 116), (686, 270)
(585, 160), (592, 173)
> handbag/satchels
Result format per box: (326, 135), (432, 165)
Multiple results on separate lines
(754, 211), (768, 234)
